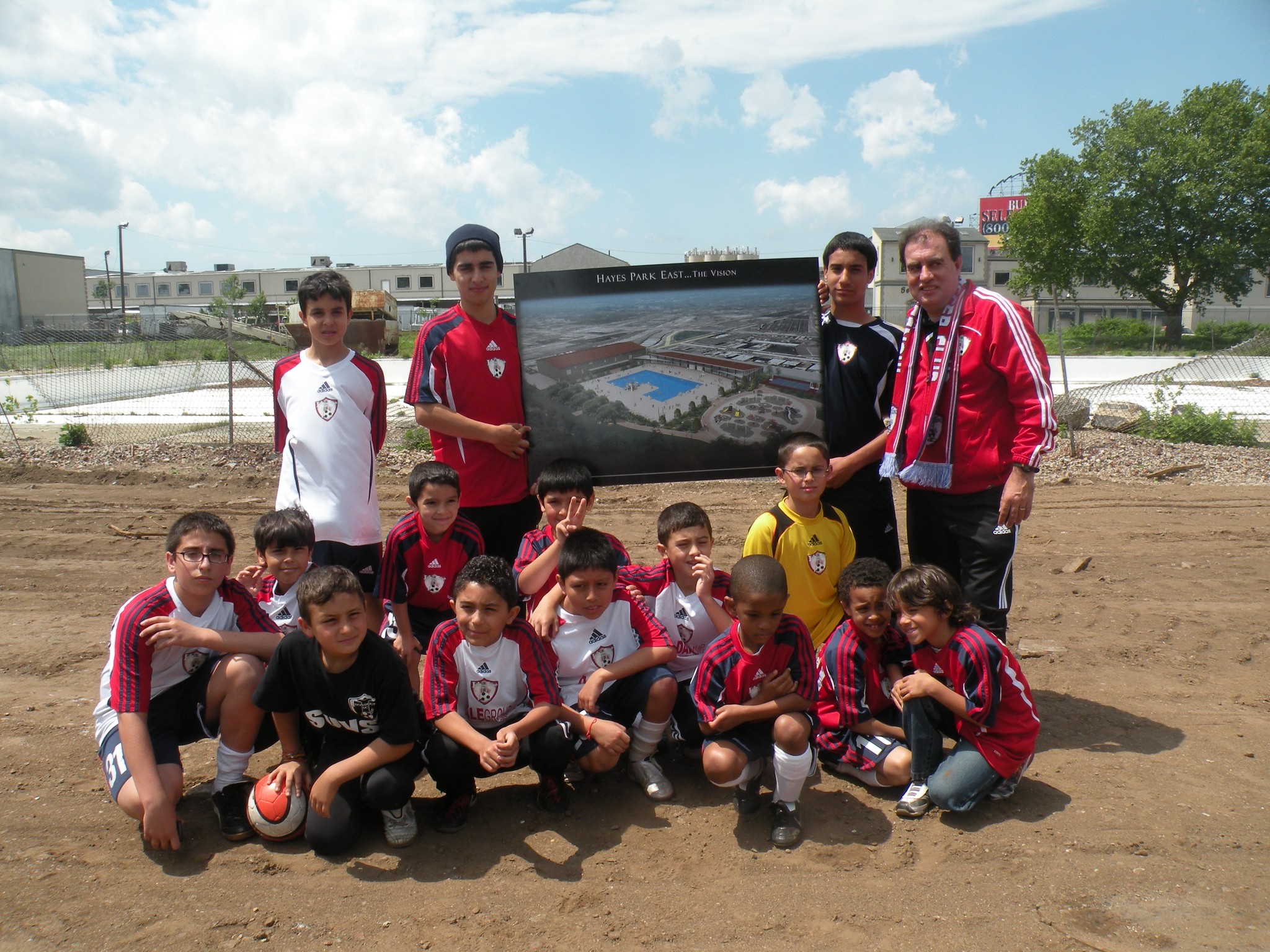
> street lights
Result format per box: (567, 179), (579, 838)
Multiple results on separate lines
(513, 226), (534, 273)
(104, 250), (113, 313)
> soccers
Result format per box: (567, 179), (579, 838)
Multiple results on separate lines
(245, 774), (308, 844)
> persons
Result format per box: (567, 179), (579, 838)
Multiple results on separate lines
(883, 564), (1040, 818)
(819, 231), (905, 581)
(528, 502), (738, 769)
(810, 557), (914, 788)
(688, 554), (818, 849)
(878, 222), (1059, 643)
(373, 459), (484, 698)
(273, 266), (389, 635)
(236, 506), (321, 669)
(403, 223), (543, 572)
(512, 458), (633, 622)
(419, 555), (576, 834)
(538, 528), (679, 803)
(741, 431), (858, 654)
(253, 565), (429, 857)
(93, 511), (286, 856)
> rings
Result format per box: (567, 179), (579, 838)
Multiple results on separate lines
(1019, 508), (1026, 511)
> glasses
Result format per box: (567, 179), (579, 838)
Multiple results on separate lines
(172, 550), (232, 564)
(780, 467), (829, 477)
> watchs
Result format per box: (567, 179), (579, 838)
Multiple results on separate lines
(1011, 462), (1039, 475)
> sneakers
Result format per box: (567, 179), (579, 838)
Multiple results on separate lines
(565, 760), (585, 782)
(985, 750), (1035, 800)
(894, 779), (933, 816)
(211, 781), (256, 842)
(537, 773), (568, 813)
(771, 799), (802, 847)
(433, 788), (477, 832)
(732, 757), (768, 815)
(381, 799), (419, 846)
(626, 750), (674, 801)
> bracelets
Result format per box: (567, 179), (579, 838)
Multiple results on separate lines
(586, 717), (600, 741)
(283, 752), (307, 762)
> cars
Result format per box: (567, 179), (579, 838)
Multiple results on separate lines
(1159, 326), (1196, 337)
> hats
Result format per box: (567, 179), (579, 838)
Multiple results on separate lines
(445, 224), (503, 275)
